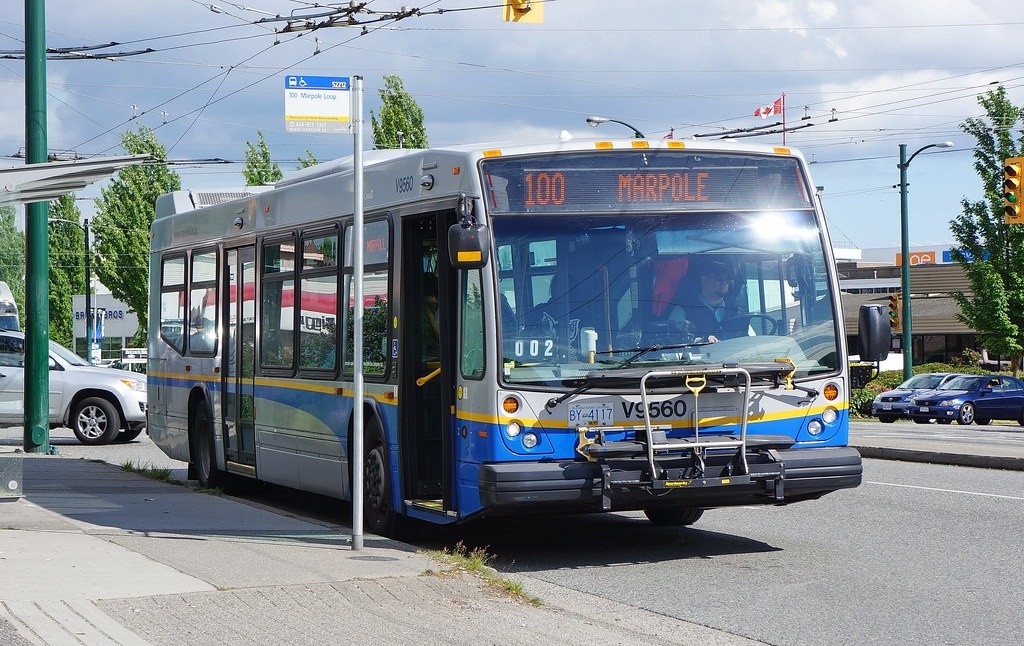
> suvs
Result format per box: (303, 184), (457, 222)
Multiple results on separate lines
(0, 326), (147, 447)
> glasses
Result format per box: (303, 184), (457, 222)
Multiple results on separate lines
(707, 275), (730, 283)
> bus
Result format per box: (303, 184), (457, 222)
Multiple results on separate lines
(144, 137), (866, 548)
(0, 281), (20, 331)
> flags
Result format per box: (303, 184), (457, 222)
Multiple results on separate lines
(754, 99), (781, 118)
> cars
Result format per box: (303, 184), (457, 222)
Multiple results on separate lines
(907, 374), (1024, 427)
(871, 373), (960, 423)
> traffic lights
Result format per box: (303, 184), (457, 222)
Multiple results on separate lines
(1004, 157), (1024, 224)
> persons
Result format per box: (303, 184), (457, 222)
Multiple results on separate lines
(425, 272), (440, 346)
(986, 380), (993, 388)
(501, 294), (519, 339)
(669, 258), (756, 344)
(524, 274), (559, 331)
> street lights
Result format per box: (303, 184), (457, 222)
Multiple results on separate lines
(896, 141), (955, 379)
(47, 218), (92, 361)
